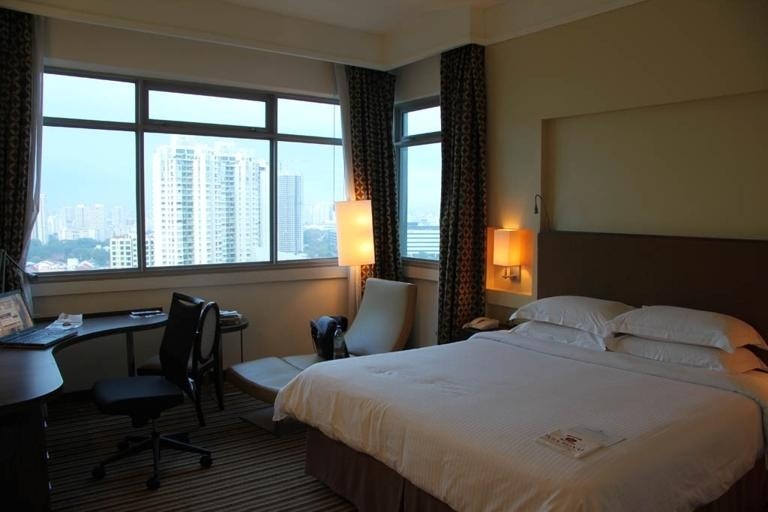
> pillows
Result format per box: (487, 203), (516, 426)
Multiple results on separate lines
(503, 291), (767, 386)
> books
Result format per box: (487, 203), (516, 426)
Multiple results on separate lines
(129, 312), (166, 320)
(537, 419), (626, 460)
(131, 310), (161, 316)
(218, 309), (243, 327)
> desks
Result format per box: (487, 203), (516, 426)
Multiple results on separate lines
(1, 308), (169, 511)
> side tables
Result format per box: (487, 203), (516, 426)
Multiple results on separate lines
(215, 319), (249, 372)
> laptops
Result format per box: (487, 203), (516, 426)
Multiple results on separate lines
(0, 289), (77, 349)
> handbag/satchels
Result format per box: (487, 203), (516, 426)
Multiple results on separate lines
(310, 315), (349, 359)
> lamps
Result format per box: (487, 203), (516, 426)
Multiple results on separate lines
(492, 231), (523, 281)
(335, 197), (376, 312)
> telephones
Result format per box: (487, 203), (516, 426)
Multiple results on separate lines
(470, 317), (500, 331)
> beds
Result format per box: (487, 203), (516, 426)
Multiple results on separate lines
(278, 234), (767, 512)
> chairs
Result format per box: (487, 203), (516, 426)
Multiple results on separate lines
(232, 275), (420, 430)
(90, 293), (224, 493)
